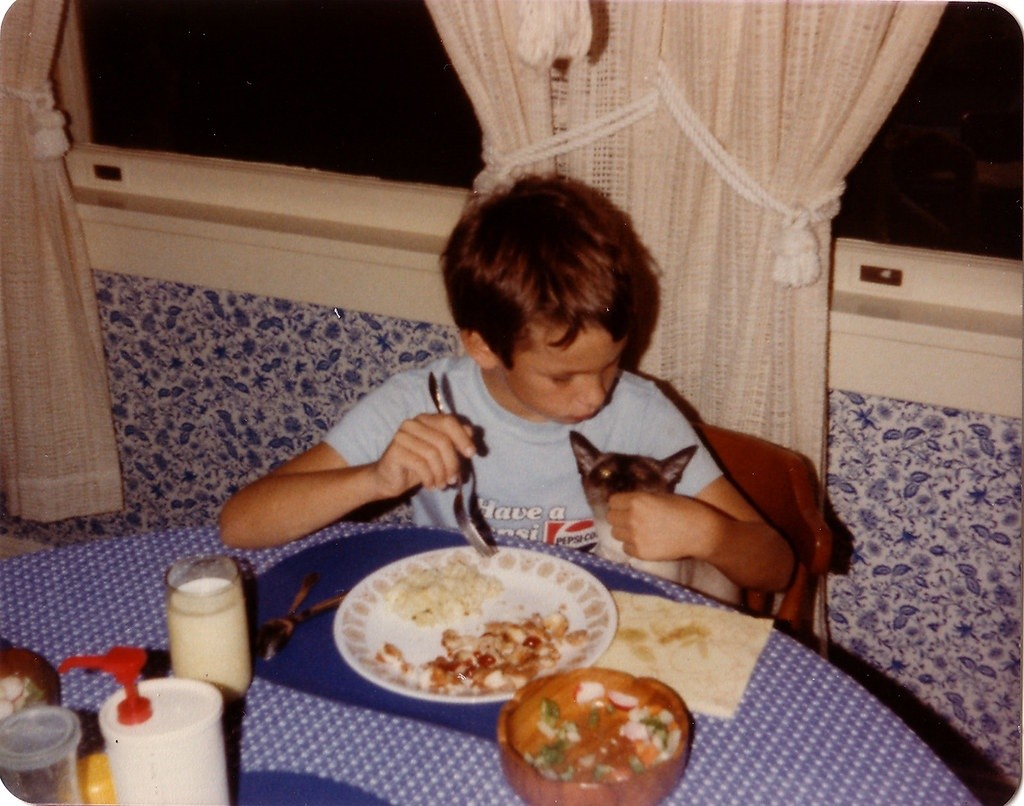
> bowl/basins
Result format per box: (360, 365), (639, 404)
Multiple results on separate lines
(495, 668), (689, 806)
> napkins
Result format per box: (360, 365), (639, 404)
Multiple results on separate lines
(593, 590), (774, 720)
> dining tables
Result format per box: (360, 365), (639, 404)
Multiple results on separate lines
(0, 521), (985, 806)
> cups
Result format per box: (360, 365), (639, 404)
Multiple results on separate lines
(164, 554), (252, 704)
(0, 705), (82, 805)
(98, 678), (230, 806)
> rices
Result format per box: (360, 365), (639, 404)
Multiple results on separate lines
(386, 560), (504, 627)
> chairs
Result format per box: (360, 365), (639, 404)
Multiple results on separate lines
(691, 422), (832, 643)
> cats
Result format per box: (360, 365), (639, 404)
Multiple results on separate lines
(569, 430), (741, 606)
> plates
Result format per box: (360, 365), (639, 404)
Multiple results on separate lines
(333, 543), (618, 705)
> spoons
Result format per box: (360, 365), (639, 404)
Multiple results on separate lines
(428, 371), (494, 557)
(249, 573), (348, 661)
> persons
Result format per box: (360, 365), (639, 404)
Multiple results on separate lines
(216, 172), (796, 615)
